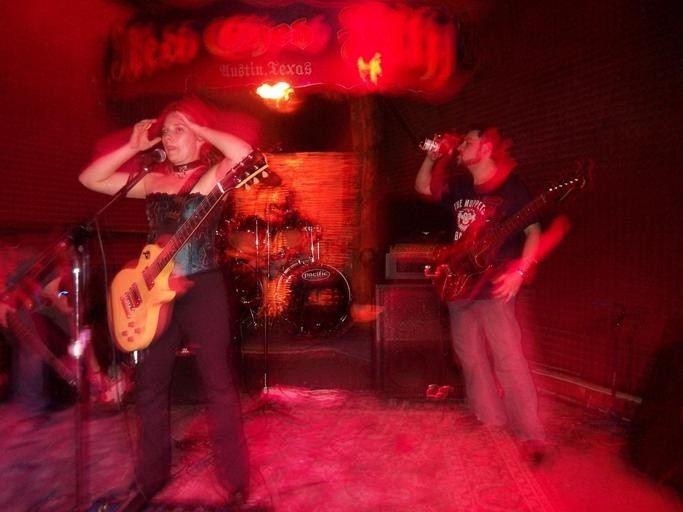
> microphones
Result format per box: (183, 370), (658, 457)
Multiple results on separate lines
(127, 148), (166, 174)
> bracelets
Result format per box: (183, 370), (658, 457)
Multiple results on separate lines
(198, 125), (207, 138)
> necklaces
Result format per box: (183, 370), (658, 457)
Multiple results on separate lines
(172, 157), (205, 181)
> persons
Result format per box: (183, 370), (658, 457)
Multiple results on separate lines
(78, 99), (255, 511)
(414, 122), (572, 470)
(0, 214), (103, 433)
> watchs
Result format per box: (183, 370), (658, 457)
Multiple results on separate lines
(515, 267), (529, 279)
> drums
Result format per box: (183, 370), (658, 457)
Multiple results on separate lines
(231, 258), (264, 322)
(274, 222), (319, 253)
(277, 257), (352, 341)
(231, 226), (268, 256)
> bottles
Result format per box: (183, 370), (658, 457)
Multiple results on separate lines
(420, 138), (457, 156)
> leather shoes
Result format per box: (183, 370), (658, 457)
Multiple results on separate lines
(129, 476), (171, 501)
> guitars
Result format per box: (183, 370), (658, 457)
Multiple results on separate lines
(104, 145), (268, 352)
(1, 253), (85, 391)
(429, 164), (592, 310)
